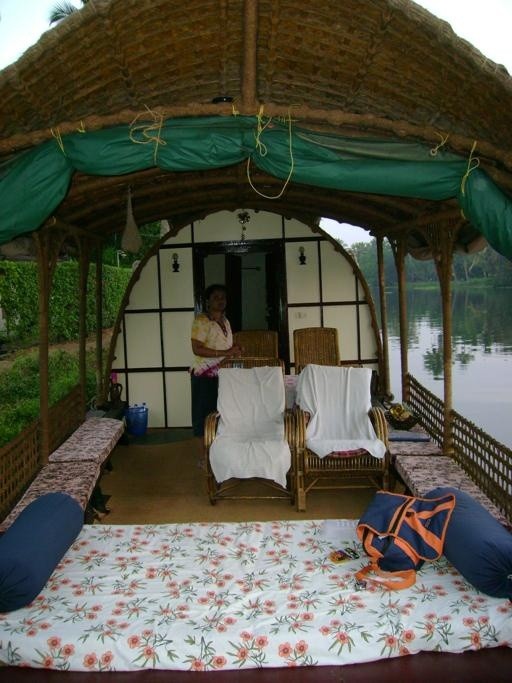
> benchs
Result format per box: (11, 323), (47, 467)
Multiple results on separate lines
(0, 413), (125, 533)
(376, 401), (509, 531)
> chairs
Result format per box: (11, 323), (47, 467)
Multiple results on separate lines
(200, 326), (392, 511)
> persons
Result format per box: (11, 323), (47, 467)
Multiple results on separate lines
(189, 284), (242, 471)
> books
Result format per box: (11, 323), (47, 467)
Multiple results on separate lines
(388, 432), (431, 442)
(326, 547), (360, 566)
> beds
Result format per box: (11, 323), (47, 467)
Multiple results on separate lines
(0, 519), (510, 682)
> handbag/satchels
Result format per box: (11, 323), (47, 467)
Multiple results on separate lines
(357, 489), (456, 572)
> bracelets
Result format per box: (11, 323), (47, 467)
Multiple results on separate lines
(215, 350), (218, 357)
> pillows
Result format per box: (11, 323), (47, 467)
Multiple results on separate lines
(0, 487), (84, 618)
(414, 485), (509, 601)
